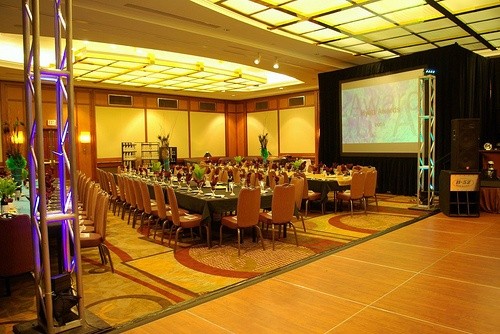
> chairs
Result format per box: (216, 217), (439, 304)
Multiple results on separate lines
(47, 161), (378, 277)
(0, 215), (48, 323)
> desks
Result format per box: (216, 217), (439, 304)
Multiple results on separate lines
(113, 173), (273, 240)
(1, 179), (40, 217)
(266, 169), (351, 212)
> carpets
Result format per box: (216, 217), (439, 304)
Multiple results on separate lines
(0, 192), (442, 334)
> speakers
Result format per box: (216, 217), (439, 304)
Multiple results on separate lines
(451, 118), (480, 172)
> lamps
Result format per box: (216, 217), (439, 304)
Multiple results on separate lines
(273, 56), (279, 69)
(253, 52), (261, 65)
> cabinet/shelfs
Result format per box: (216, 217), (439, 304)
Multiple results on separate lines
(122, 142), (177, 161)
